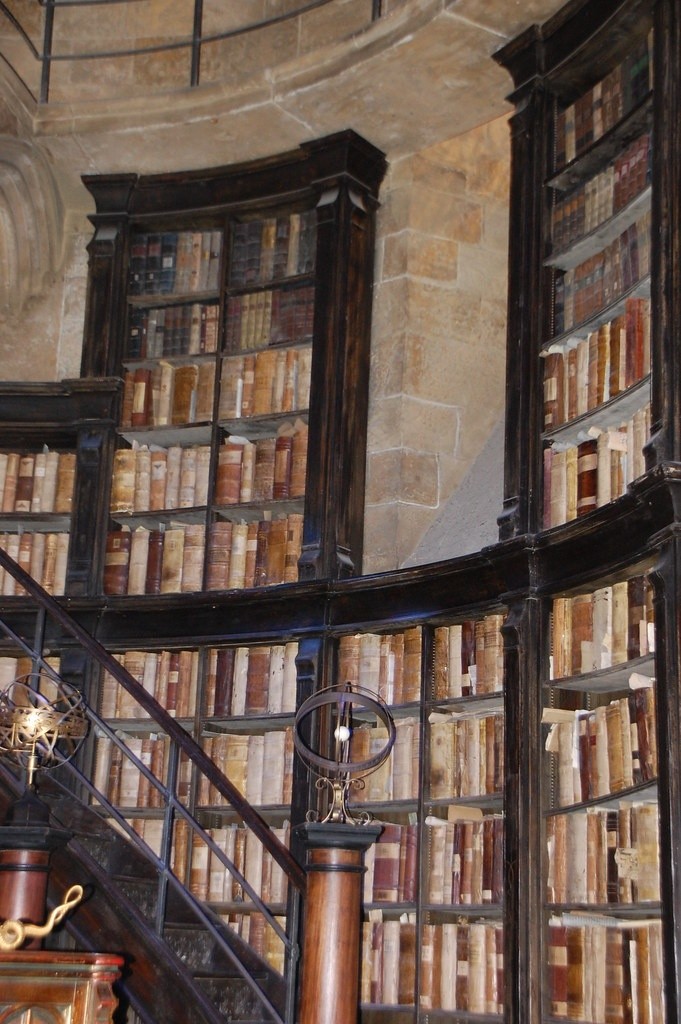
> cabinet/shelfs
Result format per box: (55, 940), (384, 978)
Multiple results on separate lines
(77, 625), (312, 1024)
(489, 0), (681, 536)
(78, 128), (392, 607)
(309, 537), (516, 1024)
(515, 465), (681, 1024)
(1, 619), (78, 802)
(0, 375), (124, 602)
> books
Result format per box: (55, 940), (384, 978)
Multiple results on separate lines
(3, 48), (677, 1024)
